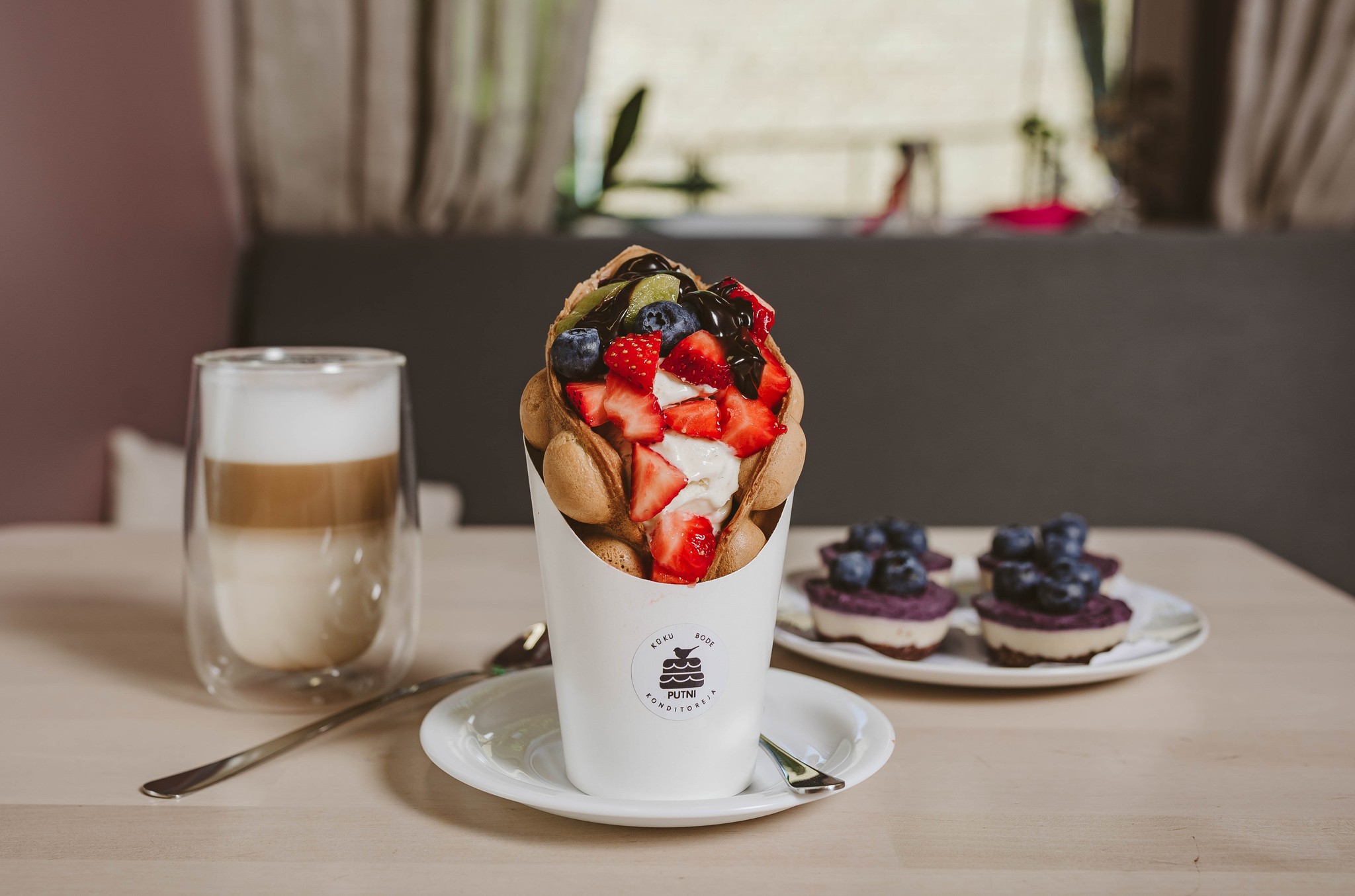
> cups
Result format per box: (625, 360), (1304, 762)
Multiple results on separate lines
(181, 345), (422, 715)
(522, 433), (795, 805)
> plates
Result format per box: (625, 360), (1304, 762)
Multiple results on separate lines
(418, 663), (896, 827)
(773, 556), (1211, 688)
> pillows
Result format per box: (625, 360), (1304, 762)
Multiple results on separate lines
(107, 429), (467, 528)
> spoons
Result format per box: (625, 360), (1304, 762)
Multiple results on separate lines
(138, 621), (552, 800)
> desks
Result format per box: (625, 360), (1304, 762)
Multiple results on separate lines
(0, 530), (1355, 896)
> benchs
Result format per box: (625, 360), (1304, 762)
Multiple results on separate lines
(239, 228), (1355, 593)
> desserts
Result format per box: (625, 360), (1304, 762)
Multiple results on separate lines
(802, 511), (1133, 667)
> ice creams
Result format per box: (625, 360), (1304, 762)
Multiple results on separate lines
(517, 243), (807, 585)
